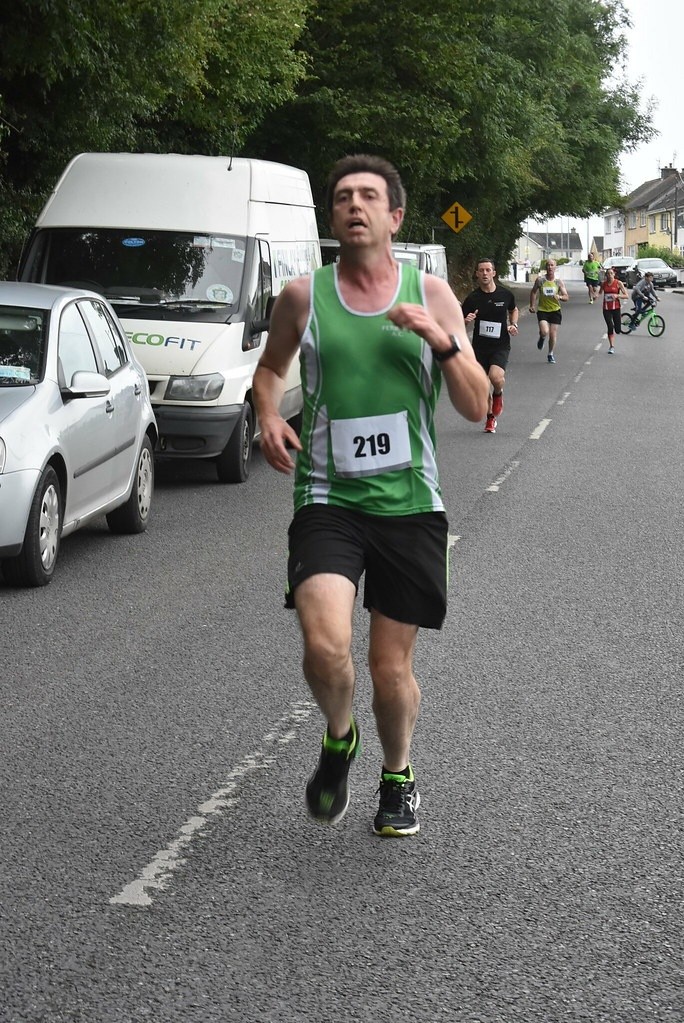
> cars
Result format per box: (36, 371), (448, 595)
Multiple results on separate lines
(598, 256), (634, 285)
(625, 258), (677, 289)
(0, 281), (160, 587)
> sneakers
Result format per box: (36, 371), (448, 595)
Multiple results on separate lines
(548, 354), (556, 363)
(370, 762), (419, 835)
(492, 389), (503, 415)
(608, 348), (615, 353)
(483, 417), (497, 434)
(537, 336), (545, 349)
(306, 713), (361, 822)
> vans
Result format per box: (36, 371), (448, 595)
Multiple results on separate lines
(15, 152), (323, 483)
(319, 240), (448, 282)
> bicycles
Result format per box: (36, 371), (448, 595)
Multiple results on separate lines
(620, 300), (665, 338)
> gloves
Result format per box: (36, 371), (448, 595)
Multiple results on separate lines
(643, 296), (648, 300)
(657, 298), (660, 302)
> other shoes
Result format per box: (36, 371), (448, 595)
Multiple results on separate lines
(628, 324), (636, 329)
(589, 300), (593, 304)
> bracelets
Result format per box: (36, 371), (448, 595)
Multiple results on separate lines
(560, 296), (562, 300)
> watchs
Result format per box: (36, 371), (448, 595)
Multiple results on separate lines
(512, 323), (518, 328)
(431, 333), (462, 362)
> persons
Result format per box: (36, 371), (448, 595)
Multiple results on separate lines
(251, 153), (492, 836)
(529, 259), (569, 364)
(627, 272), (661, 331)
(594, 268), (629, 355)
(461, 258), (519, 433)
(582, 252), (604, 304)
